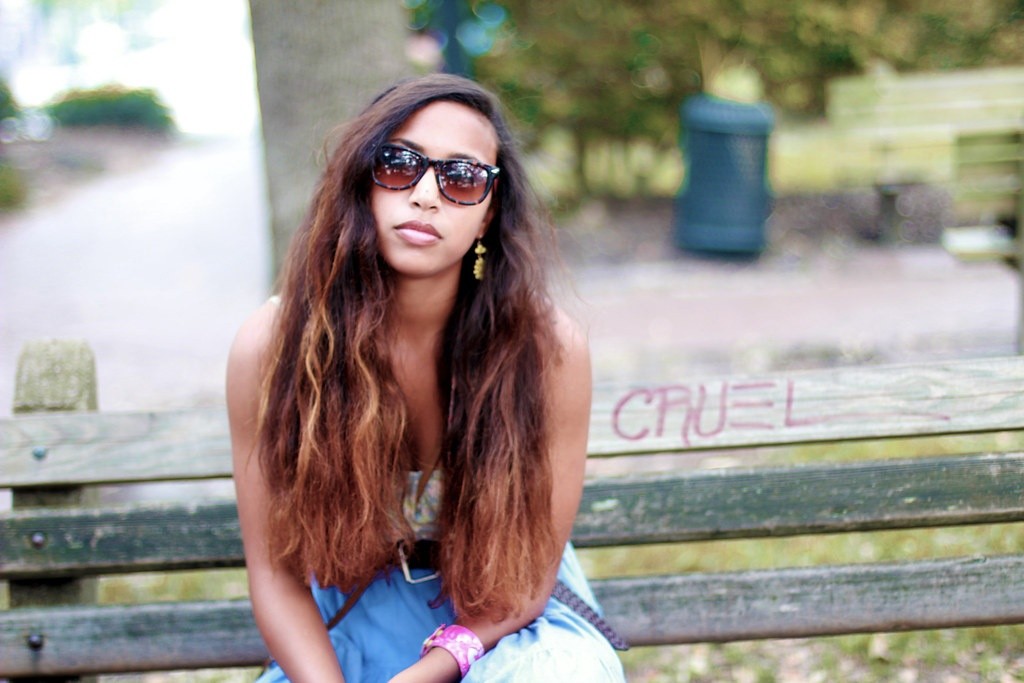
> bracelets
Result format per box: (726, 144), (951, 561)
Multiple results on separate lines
(419, 620), (486, 680)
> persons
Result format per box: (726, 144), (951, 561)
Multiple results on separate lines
(224, 72), (628, 683)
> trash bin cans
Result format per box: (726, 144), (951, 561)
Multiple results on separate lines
(671, 94), (775, 255)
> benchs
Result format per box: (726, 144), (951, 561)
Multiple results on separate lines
(0, 340), (1024, 683)
(825, 67), (1024, 240)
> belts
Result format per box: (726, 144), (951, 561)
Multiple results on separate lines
(375, 538), (443, 583)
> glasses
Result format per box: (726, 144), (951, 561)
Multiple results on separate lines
(371, 142), (500, 206)
